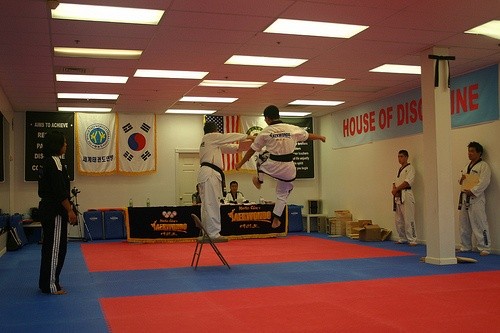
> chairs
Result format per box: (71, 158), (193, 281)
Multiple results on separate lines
(190, 214), (231, 270)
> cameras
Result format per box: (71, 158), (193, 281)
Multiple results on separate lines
(72, 187), (80, 196)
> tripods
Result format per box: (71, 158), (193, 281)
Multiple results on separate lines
(66, 196), (93, 240)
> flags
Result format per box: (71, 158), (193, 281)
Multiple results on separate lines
(238, 116), (268, 174)
(116, 112), (156, 176)
(75, 112), (119, 176)
(204, 115), (240, 173)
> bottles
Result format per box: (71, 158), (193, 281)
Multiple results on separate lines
(147, 198), (150, 207)
(193, 196), (196, 205)
(129, 198), (133, 207)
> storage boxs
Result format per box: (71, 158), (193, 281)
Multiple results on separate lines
(329, 210), (392, 241)
(308, 200), (319, 214)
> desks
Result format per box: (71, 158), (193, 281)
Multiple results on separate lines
(24, 222), (42, 245)
(304, 214), (328, 234)
(128, 204), (288, 243)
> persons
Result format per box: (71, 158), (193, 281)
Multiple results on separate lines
(38, 132), (76, 296)
(391, 150), (417, 246)
(235, 106), (325, 227)
(456, 141), (491, 256)
(197, 121), (256, 240)
(224, 181), (246, 203)
(192, 184), (201, 205)
